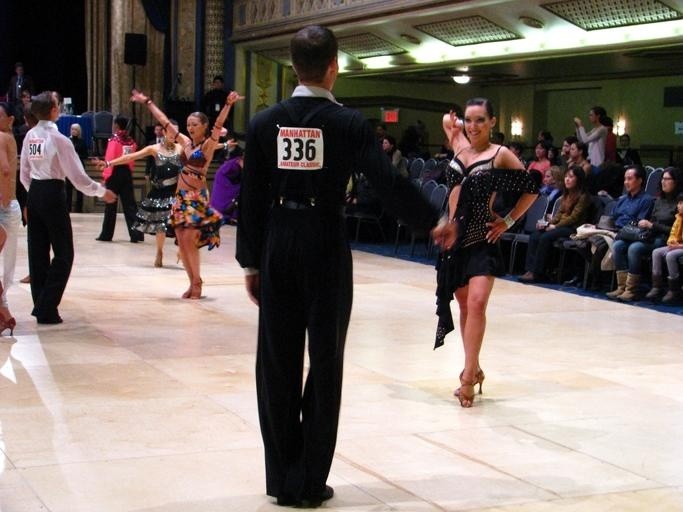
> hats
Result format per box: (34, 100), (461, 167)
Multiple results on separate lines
(596, 213), (620, 232)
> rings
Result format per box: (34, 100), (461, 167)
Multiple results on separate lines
(493, 234), (496, 237)
(496, 237), (498, 240)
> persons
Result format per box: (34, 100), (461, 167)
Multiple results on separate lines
(20, 90), (118, 324)
(236, 25), (456, 507)
(130, 88), (247, 299)
(377, 126), (409, 179)
(433, 96), (541, 409)
(8, 62), (61, 227)
(96, 113), (144, 243)
(573, 107), (617, 166)
(68, 122), (88, 212)
(491, 130), (683, 305)
(96, 119), (239, 266)
(213, 147), (245, 219)
(0, 101), (22, 336)
(146, 123), (166, 182)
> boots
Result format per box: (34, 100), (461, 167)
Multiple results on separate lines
(644, 273), (666, 300)
(660, 272), (683, 306)
(615, 271), (643, 303)
(604, 268), (629, 301)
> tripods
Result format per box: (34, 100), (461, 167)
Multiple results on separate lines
(125, 65), (150, 151)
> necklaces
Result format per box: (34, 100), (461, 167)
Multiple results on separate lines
(159, 139), (175, 157)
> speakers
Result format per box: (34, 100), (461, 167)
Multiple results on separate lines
(124, 33), (148, 66)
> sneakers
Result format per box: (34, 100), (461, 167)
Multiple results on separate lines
(562, 273), (585, 289)
(516, 270), (548, 284)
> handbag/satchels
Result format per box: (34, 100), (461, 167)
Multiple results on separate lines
(614, 220), (657, 244)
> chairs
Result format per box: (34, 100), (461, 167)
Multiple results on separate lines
(55, 109), (131, 157)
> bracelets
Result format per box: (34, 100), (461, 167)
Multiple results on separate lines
(225, 101), (233, 107)
(104, 160), (112, 168)
(504, 214), (515, 229)
(224, 142), (229, 151)
(143, 97), (153, 105)
(458, 118), (463, 125)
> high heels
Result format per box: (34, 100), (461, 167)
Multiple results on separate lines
(180, 276), (204, 301)
(0, 316), (17, 337)
(452, 362), (485, 409)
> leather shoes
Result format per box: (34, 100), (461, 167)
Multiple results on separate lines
(275, 485), (335, 509)
(130, 237), (143, 243)
(20, 275), (30, 283)
(95, 237), (113, 241)
